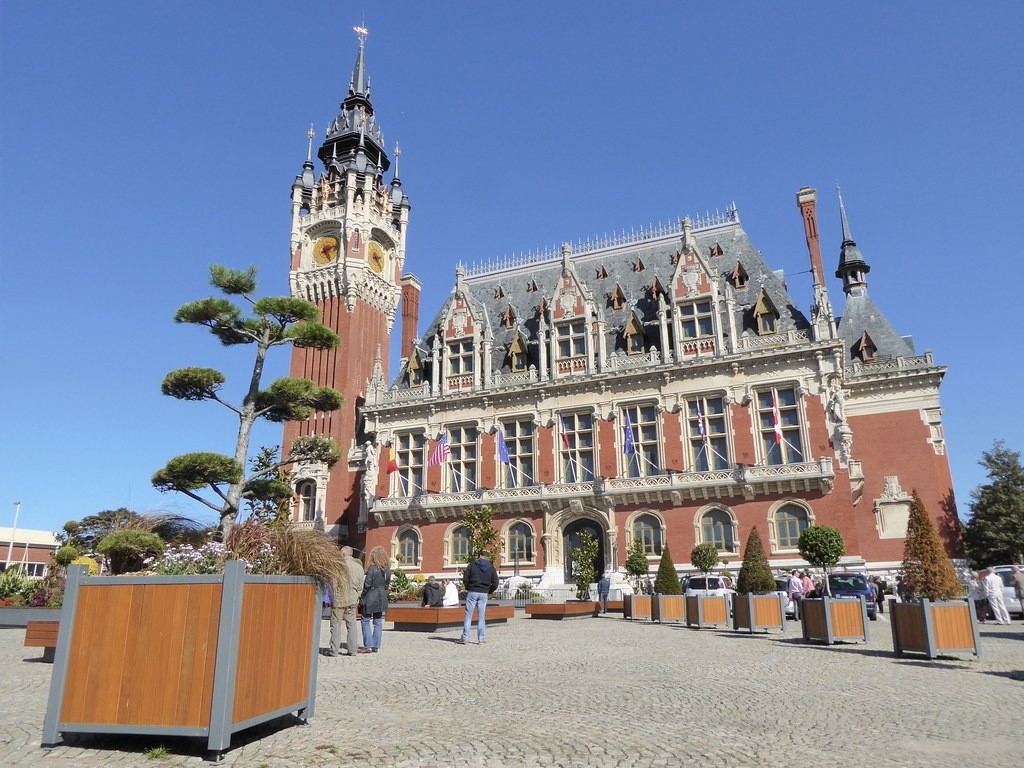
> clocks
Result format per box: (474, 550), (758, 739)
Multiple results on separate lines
(368, 242), (385, 274)
(310, 235), (340, 265)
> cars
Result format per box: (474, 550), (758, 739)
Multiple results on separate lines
(765, 578), (800, 616)
(680, 573), (737, 612)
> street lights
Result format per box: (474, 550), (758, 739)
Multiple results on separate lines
(4, 501), (22, 572)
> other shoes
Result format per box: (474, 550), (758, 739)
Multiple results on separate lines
(1021, 623), (1024, 625)
(372, 647), (378, 652)
(995, 623), (1001, 625)
(339, 652), (352, 656)
(455, 639), (467, 645)
(479, 639), (487, 644)
(358, 647), (372, 653)
(978, 619), (986, 624)
(322, 650), (332, 656)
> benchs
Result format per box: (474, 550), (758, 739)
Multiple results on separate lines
(385, 604), (515, 633)
(525, 602), (600, 620)
(601, 599), (624, 612)
(25, 616), (61, 663)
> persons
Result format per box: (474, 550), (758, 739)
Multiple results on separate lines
(325, 546), (364, 657)
(1010, 565), (1024, 625)
(789, 570), (818, 621)
(969, 567), (1011, 625)
(598, 574), (609, 614)
(896, 576), (906, 603)
(421, 576), (443, 608)
(440, 578), (459, 606)
(365, 441), (375, 471)
(454, 551), (499, 645)
(824, 385), (845, 423)
(868, 575), (885, 621)
(358, 546), (391, 653)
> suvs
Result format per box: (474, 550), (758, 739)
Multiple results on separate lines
(818, 572), (877, 621)
(987, 564), (1024, 620)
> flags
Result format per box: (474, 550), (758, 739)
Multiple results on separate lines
(559, 404), (570, 449)
(623, 408), (634, 455)
(428, 433), (451, 466)
(498, 427), (510, 463)
(386, 447), (400, 474)
(696, 396), (706, 444)
(771, 387), (783, 445)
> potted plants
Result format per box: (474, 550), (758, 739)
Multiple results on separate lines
(731, 525), (787, 634)
(39, 262), (346, 762)
(623, 536), (651, 621)
(685, 543), (731, 629)
(454, 502), (506, 600)
(653, 539), (687, 624)
(566, 529), (600, 603)
(797, 523), (870, 646)
(887, 487), (985, 659)
